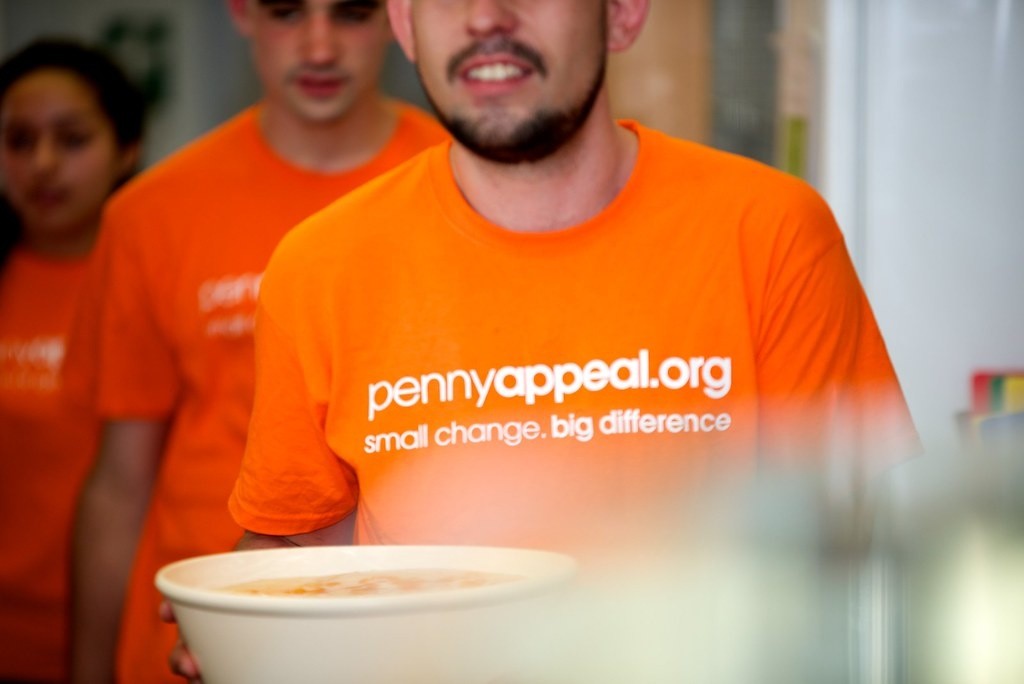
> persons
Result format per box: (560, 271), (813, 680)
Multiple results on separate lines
(161, 0), (924, 684)
(0, 39), (143, 684)
(63, 1), (457, 516)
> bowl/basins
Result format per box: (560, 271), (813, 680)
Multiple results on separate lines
(155, 544), (573, 684)
